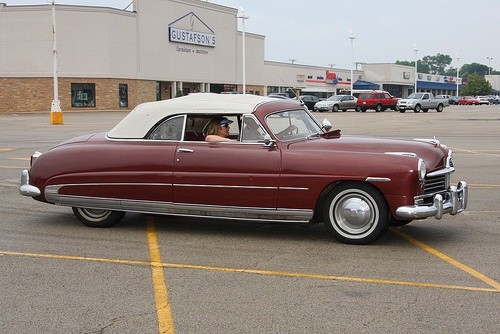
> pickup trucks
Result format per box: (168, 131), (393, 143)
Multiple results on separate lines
(397, 91), (450, 113)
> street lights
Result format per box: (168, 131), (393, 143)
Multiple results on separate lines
(235, 5), (251, 94)
(413, 48), (420, 93)
(486, 56), (492, 75)
(456, 57), (460, 96)
(347, 30), (357, 96)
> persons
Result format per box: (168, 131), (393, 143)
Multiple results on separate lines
(194, 117), (236, 142)
(243, 117), (295, 140)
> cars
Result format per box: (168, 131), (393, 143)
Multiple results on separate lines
(435, 94), (500, 105)
(267, 91), (328, 102)
(19, 92), (469, 246)
(314, 94), (358, 112)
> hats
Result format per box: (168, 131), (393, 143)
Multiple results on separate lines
(211, 116), (234, 126)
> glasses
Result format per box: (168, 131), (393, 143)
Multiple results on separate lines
(222, 124), (228, 128)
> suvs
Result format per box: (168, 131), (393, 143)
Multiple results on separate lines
(356, 90), (403, 112)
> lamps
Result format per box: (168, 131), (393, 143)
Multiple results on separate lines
(165, 88), (167, 91)
(193, 88), (196, 92)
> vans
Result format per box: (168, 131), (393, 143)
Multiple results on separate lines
(292, 94), (322, 112)
